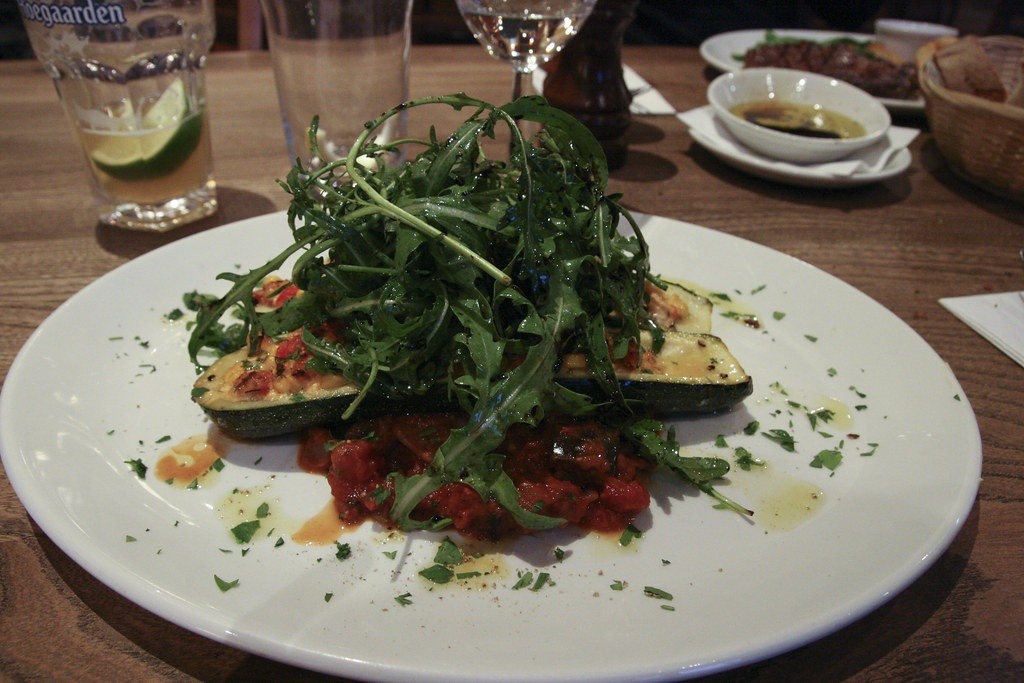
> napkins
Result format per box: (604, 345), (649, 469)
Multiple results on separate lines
(532, 62), (676, 115)
(939, 290), (1024, 367)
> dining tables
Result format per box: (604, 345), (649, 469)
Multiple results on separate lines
(0, 43), (1023, 683)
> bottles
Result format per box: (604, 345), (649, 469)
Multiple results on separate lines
(540, 1), (632, 170)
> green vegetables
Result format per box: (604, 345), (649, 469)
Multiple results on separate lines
(732, 29), (890, 61)
(107, 93), (878, 600)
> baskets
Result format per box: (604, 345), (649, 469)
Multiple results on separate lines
(917, 36), (1024, 197)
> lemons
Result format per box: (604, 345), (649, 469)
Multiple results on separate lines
(90, 77), (202, 181)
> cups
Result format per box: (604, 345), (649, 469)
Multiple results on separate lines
(16, 0), (220, 234)
(874, 17), (959, 62)
(259, 1), (416, 196)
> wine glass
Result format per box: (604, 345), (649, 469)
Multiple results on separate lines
(455, 0), (597, 161)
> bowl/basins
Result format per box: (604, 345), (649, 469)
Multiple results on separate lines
(708, 66), (893, 166)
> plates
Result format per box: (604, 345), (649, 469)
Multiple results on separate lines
(689, 110), (914, 186)
(697, 28), (925, 116)
(0, 208), (984, 682)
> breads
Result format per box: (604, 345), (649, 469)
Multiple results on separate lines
(932, 35), (1024, 108)
